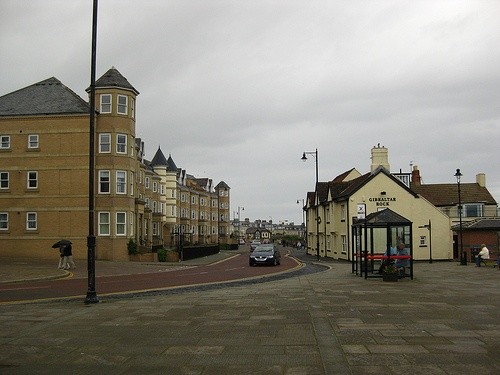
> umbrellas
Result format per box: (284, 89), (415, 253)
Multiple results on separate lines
(51, 239), (72, 248)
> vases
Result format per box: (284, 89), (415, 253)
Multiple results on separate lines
(382, 274), (398, 281)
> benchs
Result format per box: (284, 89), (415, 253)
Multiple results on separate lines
(481, 259), (488, 266)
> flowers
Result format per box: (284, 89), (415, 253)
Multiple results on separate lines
(379, 265), (402, 274)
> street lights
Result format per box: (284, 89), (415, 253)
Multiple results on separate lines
(296, 199), (306, 249)
(300, 148), (319, 261)
(453, 168), (463, 265)
(233, 211), (238, 238)
(237, 205), (245, 239)
(269, 215), (272, 235)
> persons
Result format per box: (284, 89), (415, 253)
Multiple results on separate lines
(284, 240), (305, 249)
(379, 247), (394, 273)
(475, 244), (490, 266)
(395, 243), (408, 271)
(59, 242), (76, 269)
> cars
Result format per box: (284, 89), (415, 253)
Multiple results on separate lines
(248, 243), (281, 267)
(249, 239), (271, 252)
(240, 239), (245, 245)
